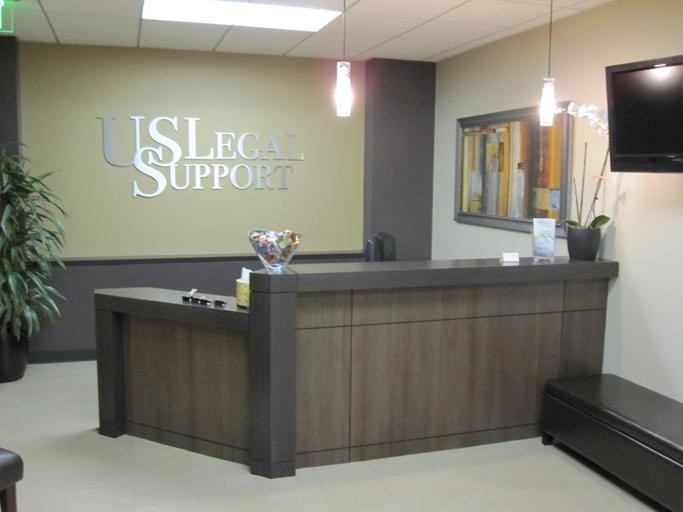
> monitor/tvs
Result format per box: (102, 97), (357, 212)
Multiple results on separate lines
(605, 52), (683, 173)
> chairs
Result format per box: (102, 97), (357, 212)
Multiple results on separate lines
(366, 232), (397, 262)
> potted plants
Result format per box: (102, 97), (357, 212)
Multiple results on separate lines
(0, 140), (71, 383)
(556, 215), (610, 261)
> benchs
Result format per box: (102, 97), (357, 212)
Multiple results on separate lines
(540, 373), (683, 512)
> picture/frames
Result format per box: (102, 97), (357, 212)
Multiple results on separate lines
(453, 100), (575, 238)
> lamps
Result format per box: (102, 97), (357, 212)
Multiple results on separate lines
(336, 0), (352, 117)
(539, 0), (556, 127)
